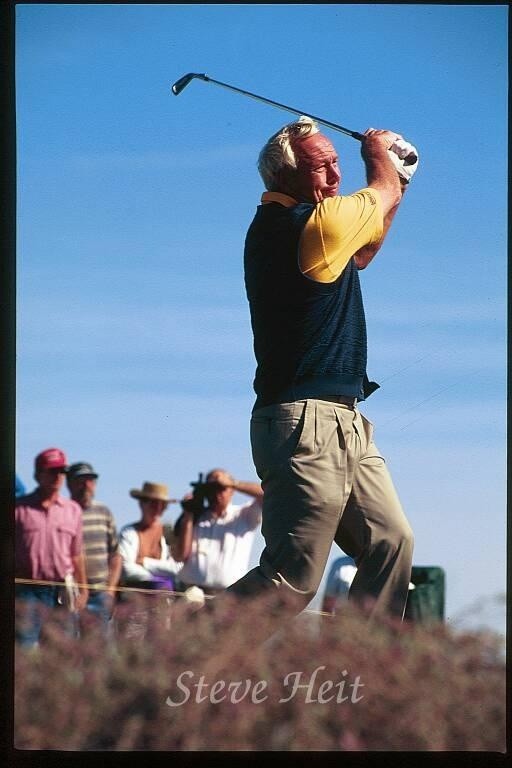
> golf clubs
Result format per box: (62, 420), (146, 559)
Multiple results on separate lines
(172, 72), (417, 164)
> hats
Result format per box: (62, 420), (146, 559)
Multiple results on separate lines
(35, 449), (178, 503)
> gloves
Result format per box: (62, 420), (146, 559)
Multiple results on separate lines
(386, 137), (419, 185)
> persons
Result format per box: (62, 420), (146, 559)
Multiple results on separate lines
(117, 481), (185, 671)
(169, 466), (265, 618)
(12, 445), (88, 660)
(66, 462), (124, 664)
(177, 114), (418, 692)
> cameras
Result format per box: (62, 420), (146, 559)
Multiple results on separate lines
(180, 473), (225, 522)
